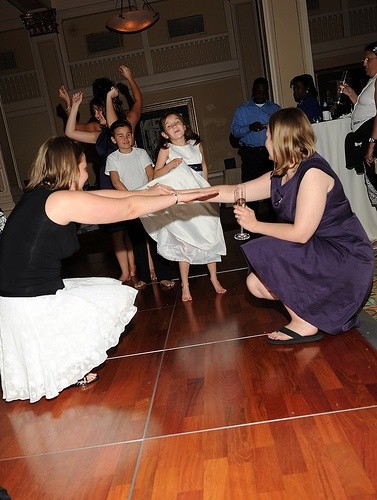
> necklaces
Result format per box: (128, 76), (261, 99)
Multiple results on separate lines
(299, 100), (303, 105)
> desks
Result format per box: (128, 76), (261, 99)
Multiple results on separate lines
(311, 116), (377, 244)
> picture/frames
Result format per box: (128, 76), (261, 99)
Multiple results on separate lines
(123, 96), (200, 166)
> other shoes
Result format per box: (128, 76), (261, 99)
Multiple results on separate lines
(73, 373), (98, 385)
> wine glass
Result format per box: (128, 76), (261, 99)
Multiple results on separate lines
(234, 183), (250, 240)
(333, 71), (352, 105)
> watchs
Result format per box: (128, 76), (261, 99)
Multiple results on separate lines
(369, 137), (377, 144)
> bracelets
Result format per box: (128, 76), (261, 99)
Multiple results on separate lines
(63, 106), (71, 112)
(172, 192), (179, 204)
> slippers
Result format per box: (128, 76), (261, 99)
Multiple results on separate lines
(267, 326), (324, 344)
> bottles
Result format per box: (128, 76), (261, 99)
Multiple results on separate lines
(322, 99), (330, 121)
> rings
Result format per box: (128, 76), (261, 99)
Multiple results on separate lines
(257, 130), (259, 132)
(117, 92), (119, 95)
(125, 66), (128, 70)
(258, 129), (259, 130)
(237, 215), (239, 219)
(370, 159), (371, 160)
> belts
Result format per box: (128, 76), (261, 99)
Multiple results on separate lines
(247, 146), (265, 151)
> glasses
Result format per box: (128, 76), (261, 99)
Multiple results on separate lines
(361, 57), (377, 62)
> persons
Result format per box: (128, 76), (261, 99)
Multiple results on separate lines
(146, 125), (375, 345)
(0, 134), (220, 404)
(230, 42), (377, 250)
(58, 63), (228, 301)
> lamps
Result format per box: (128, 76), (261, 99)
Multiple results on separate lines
(106, 0), (160, 34)
